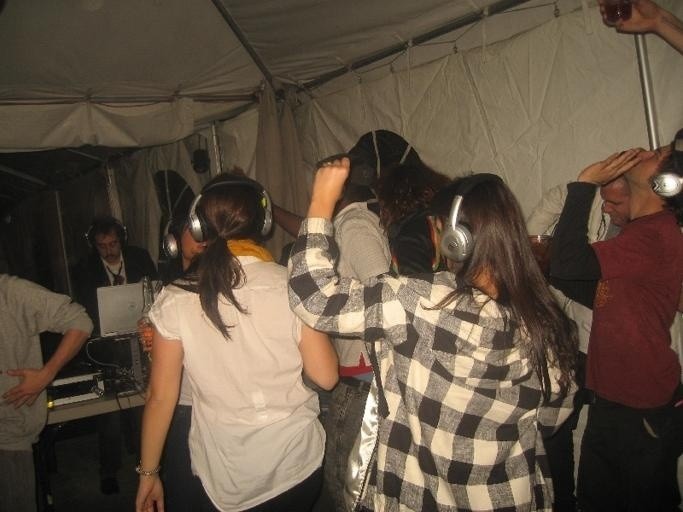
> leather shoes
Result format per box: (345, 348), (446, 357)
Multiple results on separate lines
(96, 466), (122, 496)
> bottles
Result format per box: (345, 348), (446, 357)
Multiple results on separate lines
(137, 274), (161, 354)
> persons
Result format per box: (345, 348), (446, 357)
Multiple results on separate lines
(69, 219), (160, 363)
(0, 271), (95, 510)
(597, 0), (683, 51)
(134, 130), (682, 511)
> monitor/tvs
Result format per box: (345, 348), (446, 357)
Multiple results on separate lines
(96, 281), (164, 338)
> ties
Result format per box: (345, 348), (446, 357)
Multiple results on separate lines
(106, 262), (125, 287)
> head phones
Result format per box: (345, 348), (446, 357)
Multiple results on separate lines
(439, 173), (502, 263)
(83, 219), (128, 246)
(655, 128), (683, 198)
(138, 276), (155, 331)
(188, 177), (273, 242)
(161, 220), (182, 259)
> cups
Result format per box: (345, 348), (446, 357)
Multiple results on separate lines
(525, 233), (553, 288)
